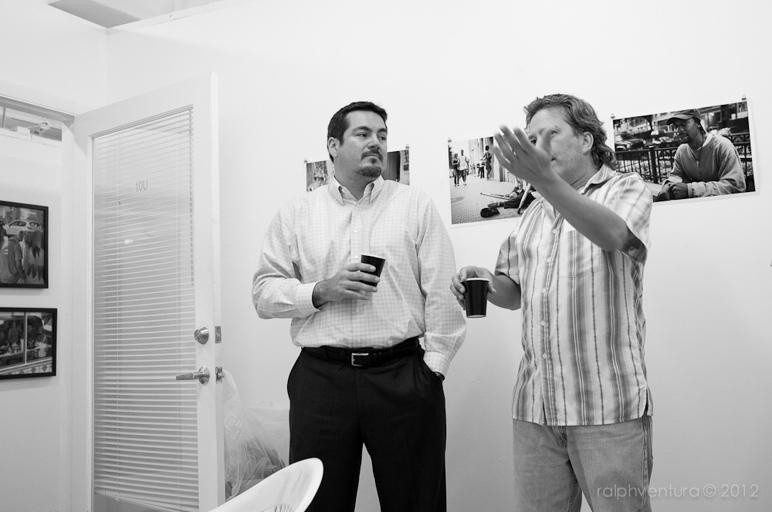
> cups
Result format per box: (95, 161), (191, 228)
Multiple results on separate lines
(358, 254), (386, 292)
(460, 277), (489, 319)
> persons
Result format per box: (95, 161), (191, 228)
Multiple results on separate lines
(652, 108), (747, 202)
(446, 91), (659, 512)
(0, 217), (54, 370)
(446, 144), (493, 188)
(247, 100), (471, 511)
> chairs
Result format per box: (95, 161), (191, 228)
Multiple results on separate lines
(201, 457), (325, 512)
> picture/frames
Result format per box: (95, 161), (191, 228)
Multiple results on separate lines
(302, 96), (760, 228)
(0, 199), (59, 381)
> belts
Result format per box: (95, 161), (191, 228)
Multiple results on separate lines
(318, 339), (418, 368)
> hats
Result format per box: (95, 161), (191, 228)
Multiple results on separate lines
(665, 110), (701, 125)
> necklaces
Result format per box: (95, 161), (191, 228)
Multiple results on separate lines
(688, 131), (706, 170)
